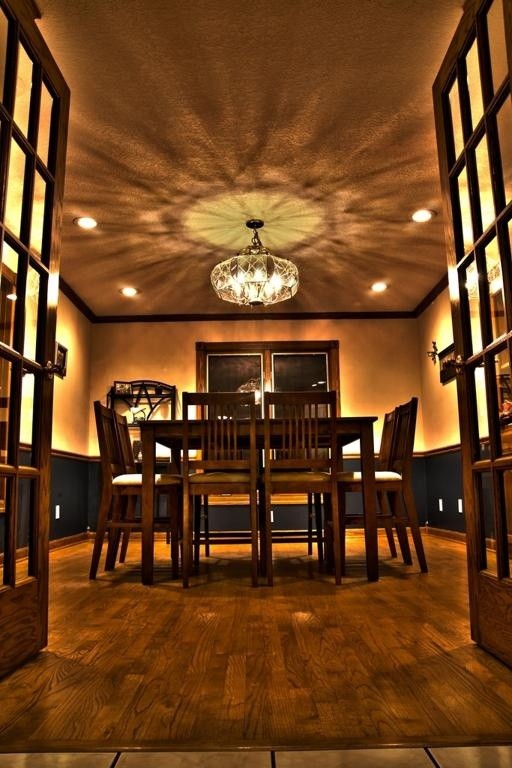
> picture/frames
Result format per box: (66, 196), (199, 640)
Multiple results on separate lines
(111, 379), (134, 396)
(54, 340), (68, 377)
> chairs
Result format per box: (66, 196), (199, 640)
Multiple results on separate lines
(86, 396), (186, 585)
(311, 395), (430, 577)
(262, 388), (343, 587)
(181, 388), (259, 587)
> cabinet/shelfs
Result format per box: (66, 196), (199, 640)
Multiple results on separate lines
(104, 378), (177, 460)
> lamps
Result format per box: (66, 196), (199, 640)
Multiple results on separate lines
(208, 216), (302, 308)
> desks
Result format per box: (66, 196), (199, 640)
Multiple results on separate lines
(136, 415), (380, 586)
